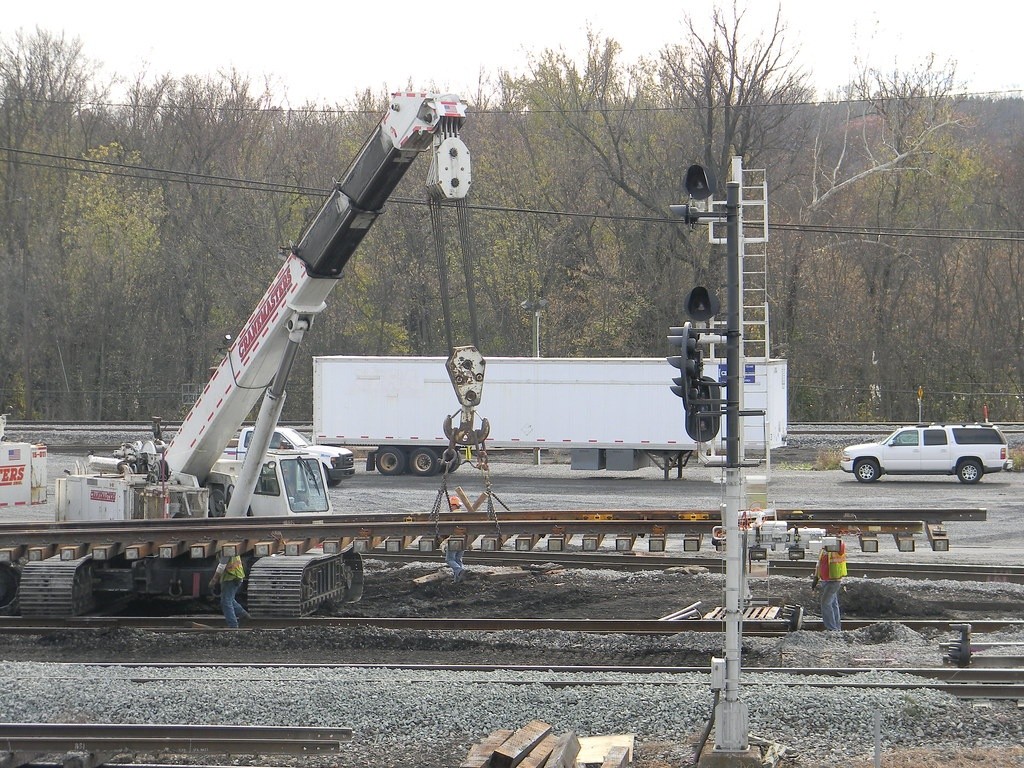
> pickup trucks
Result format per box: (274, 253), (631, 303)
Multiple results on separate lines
(222, 424), (355, 488)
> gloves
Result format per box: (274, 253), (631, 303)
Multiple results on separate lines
(812, 576), (818, 590)
(208, 580), (216, 589)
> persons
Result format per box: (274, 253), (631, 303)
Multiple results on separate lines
(207, 554), (251, 629)
(811, 536), (847, 631)
(444, 495), (471, 584)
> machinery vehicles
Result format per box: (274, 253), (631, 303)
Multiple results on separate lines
(16, 90), (477, 622)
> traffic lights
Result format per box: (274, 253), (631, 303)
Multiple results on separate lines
(670, 330), (708, 410)
(682, 376), (724, 441)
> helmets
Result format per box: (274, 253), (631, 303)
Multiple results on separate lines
(450, 496), (461, 506)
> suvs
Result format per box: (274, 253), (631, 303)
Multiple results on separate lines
(839, 425), (1012, 483)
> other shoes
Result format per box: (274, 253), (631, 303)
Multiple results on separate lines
(454, 568), (466, 583)
